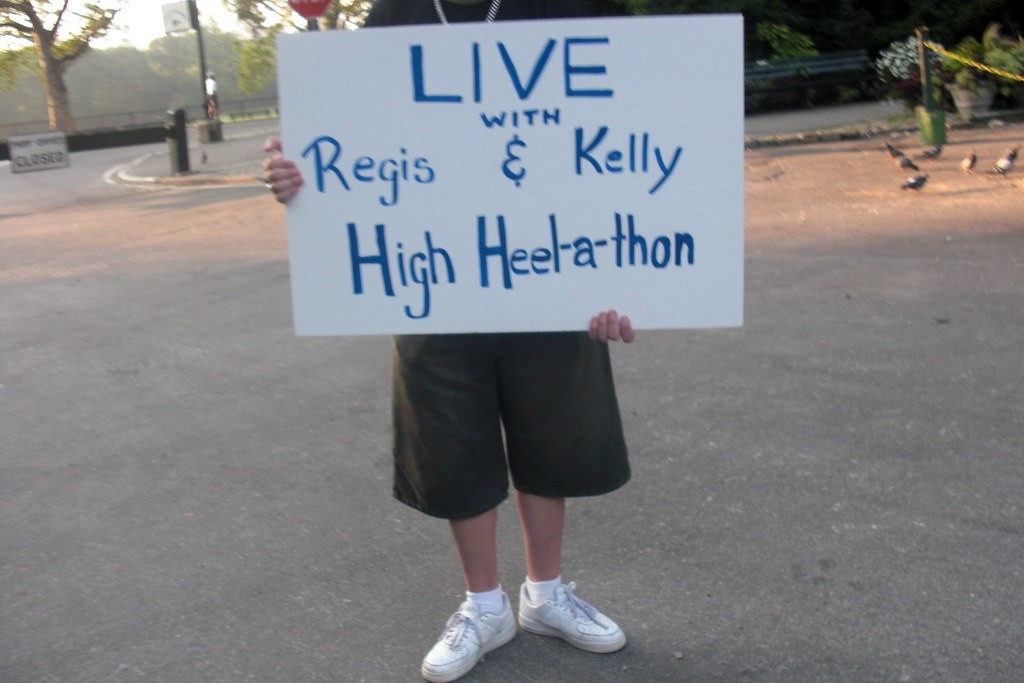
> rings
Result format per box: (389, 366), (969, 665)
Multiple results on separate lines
(263, 181), (271, 188)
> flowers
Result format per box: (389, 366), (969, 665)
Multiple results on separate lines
(875, 37), (945, 100)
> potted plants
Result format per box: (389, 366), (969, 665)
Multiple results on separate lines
(941, 23), (1024, 115)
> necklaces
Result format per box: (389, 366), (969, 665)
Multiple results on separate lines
(433, 0), (500, 24)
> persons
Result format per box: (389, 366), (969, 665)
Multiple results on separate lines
(203, 72), (223, 124)
(253, 0), (639, 682)
(881, 59), (953, 112)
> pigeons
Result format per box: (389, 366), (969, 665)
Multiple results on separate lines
(914, 144), (943, 164)
(884, 142), (905, 159)
(957, 148), (977, 176)
(892, 154), (919, 173)
(982, 148), (1018, 179)
(899, 169), (929, 194)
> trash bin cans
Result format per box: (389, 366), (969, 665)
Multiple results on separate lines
(162, 108), (190, 177)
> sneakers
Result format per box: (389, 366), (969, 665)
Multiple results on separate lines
(518, 581), (626, 654)
(421, 592), (516, 683)
